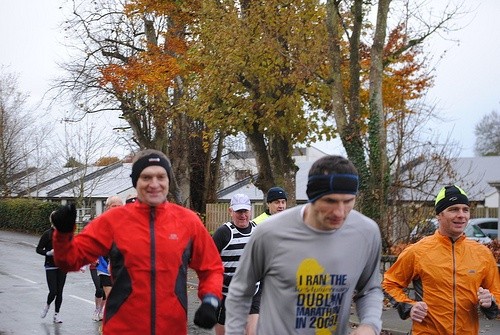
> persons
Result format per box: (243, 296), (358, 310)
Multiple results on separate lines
(252, 186), (288, 227)
(224, 155), (384, 335)
(50, 149), (224, 335)
(36, 210), (86, 324)
(89, 193), (138, 335)
(212, 193), (264, 335)
(382, 185), (500, 335)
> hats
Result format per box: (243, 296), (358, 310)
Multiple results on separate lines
(267, 187), (287, 202)
(230, 194), (251, 212)
(434, 185), (471, 215)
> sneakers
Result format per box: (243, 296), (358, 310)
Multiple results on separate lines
(41, 305), (49, 318)
(91, 309), (103, 322)
(53, 312), (62, 323)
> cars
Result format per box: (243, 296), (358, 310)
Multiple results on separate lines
(464, 218), (500, 246)
(409, 218), (440, 245)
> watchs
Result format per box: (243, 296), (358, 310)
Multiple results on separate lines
(480, 294), (497, 312)
(200, 296), (219, 311)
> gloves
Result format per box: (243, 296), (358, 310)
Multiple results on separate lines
(51, 203), (77, 233)
(193, 301), (218, 330)
